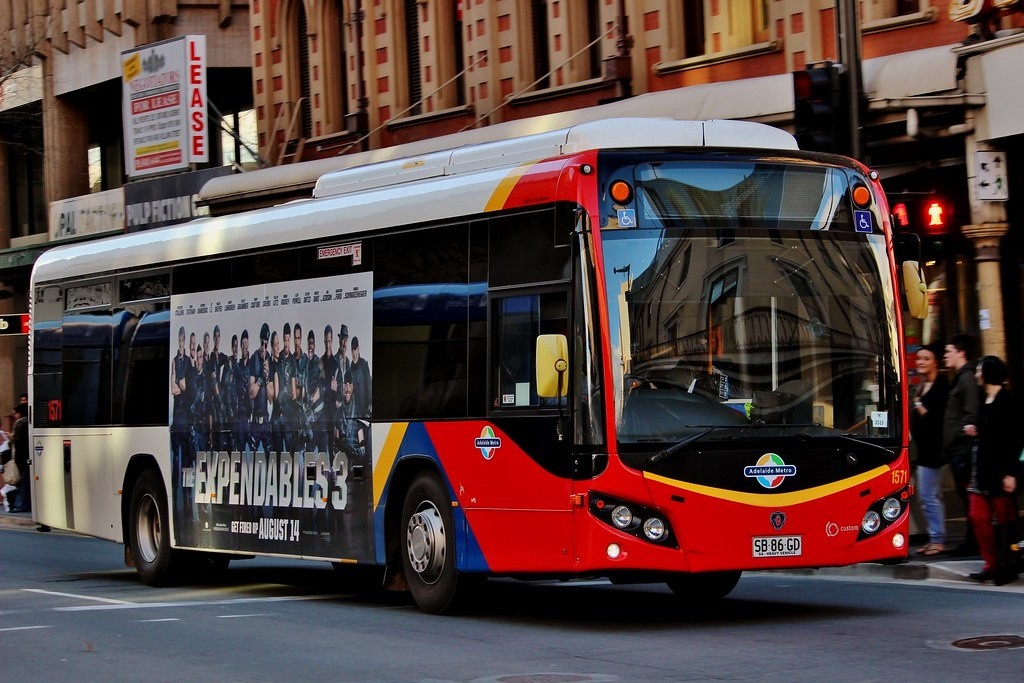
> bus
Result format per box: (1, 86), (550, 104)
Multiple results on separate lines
(26, 117), (928, 616)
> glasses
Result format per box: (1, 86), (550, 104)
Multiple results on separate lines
(261, 341), (268, 348)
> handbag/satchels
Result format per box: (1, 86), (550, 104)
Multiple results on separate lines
(3, 459), (20, 484)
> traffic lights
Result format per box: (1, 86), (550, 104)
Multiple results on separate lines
(792, 61), (841, 156)
(876, 165), (959, 263)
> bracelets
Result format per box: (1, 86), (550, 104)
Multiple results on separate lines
(914, 402), (923, 407)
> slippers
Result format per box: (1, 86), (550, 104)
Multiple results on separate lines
(917, 547), (929, 554)
(923, 547), (950, 556)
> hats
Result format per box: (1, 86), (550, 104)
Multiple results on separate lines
(241, 330), (248, 342)
(260, 323), (270, 340)
(338, 324), (349, 338)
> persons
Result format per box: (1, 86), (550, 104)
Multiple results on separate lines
(4, 394), (32, 512)
(905, 344), (952, 556)
(492, 361), (594, 408)
(938, 335), (982, 551)
(965, 354), (1024, 585)
(168, 324), (371, 535)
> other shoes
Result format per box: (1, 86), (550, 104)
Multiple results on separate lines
(970, 570), (993, 581)
